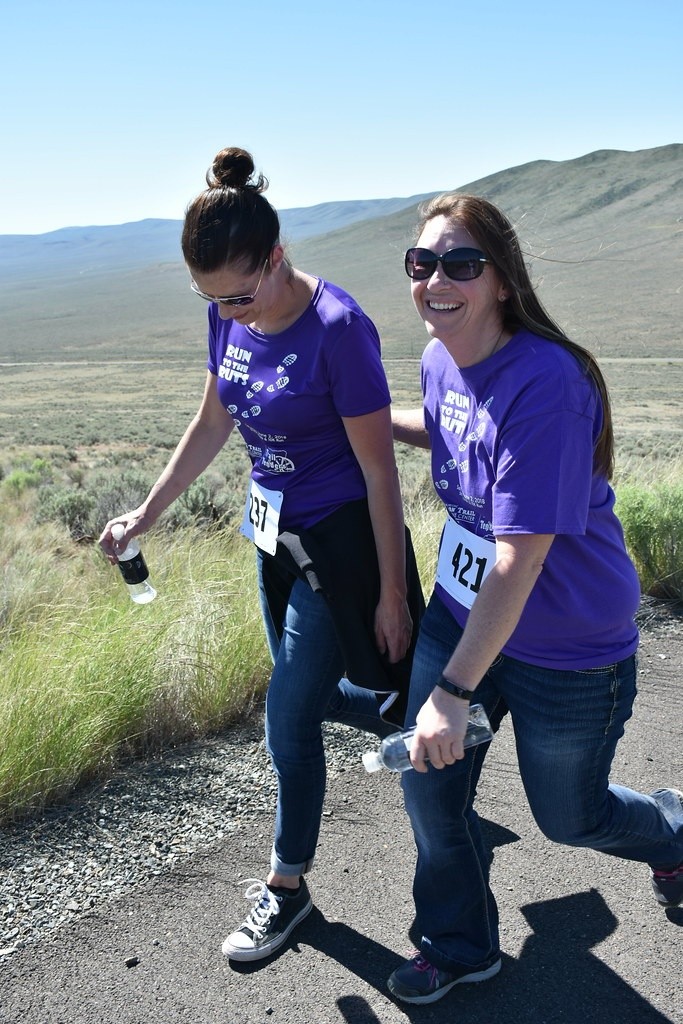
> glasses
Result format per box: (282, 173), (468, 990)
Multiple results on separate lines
(190, 256), (269, 306)
(405, 248), (497, 281)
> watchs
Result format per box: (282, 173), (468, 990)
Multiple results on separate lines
(436, 675), (475, 700)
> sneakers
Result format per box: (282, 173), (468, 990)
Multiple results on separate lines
(649, 787), (683, 907)
(388, 949), (502, 1004)
(222, 876), (313, 961)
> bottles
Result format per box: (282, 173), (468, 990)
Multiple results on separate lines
(361, 704), (495, 773)
(111, 524), (156, 604)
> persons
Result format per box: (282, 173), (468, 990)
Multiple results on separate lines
(92, 145), (494, 961)
(384, 193), (683, 1004)
